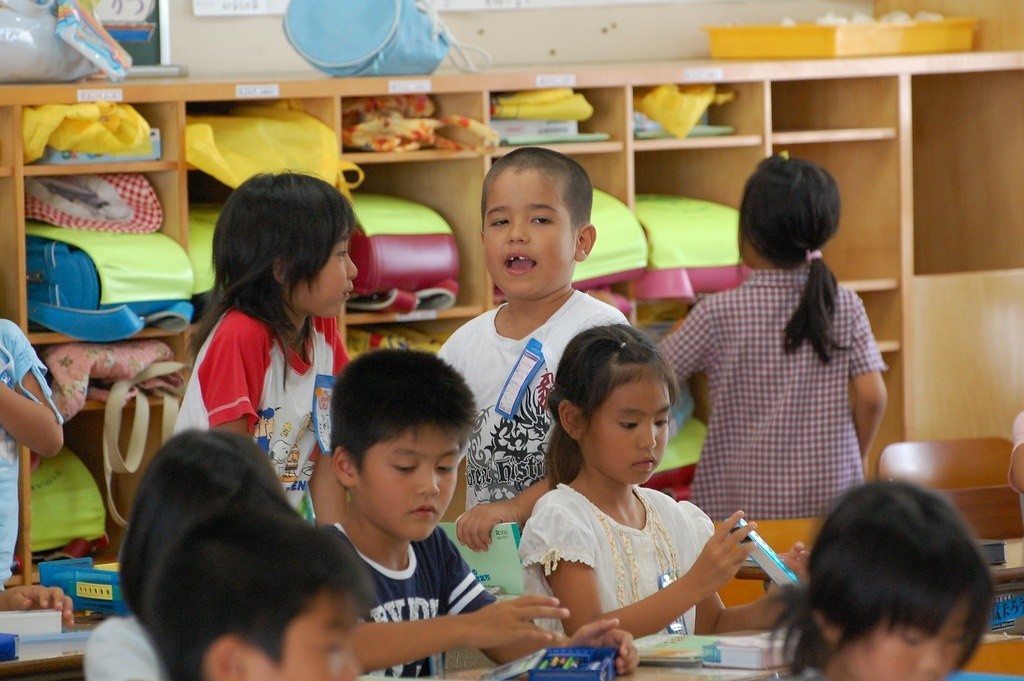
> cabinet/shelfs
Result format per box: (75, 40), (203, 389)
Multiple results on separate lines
(0, 49), (1024, 537)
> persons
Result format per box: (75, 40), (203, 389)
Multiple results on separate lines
(435, 146), (627, 551)
(171, 173), (359, 524)
(654, 154), (888, 521)
(0, 315), (378, 680)
(323, 347), (638, 681)
(515, 325), (808, 641)
(771, 479), (999, 681)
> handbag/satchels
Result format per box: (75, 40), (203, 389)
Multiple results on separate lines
(341, 95), (501, 156)
(282, 0), (491, 76)
(22, 100), (153, 164)
(185, 106), (364, 199)
(635, 85), (734, 139)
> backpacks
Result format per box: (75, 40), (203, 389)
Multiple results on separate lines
(348, 192), (461, 315)
(635, 193), (752, 305)
(495, 188), (648, 316)
(24, 223), (194, 341)
(13, 446), (110, 573)
(188, 205), (222, 324)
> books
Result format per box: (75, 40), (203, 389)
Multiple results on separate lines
(0, 525), (1022, 681)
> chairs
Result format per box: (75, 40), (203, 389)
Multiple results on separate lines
(712, 517), (824, 606)
(878, 437), (1014, 490)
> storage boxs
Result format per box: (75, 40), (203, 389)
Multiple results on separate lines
(39, 128), (162, 165)
(699, 16), (981, 59)
(490, 119), (579, 138)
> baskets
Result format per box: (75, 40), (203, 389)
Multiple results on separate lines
(37, 557), (133, 617)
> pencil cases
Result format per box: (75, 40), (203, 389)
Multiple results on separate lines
(482, 644), (618, 681)
(702, 630), (798, 669)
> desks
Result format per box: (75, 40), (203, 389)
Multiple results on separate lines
(442, 636), (1024, 681)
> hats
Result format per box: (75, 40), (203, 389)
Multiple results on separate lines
(24, 172), (163, 234)
